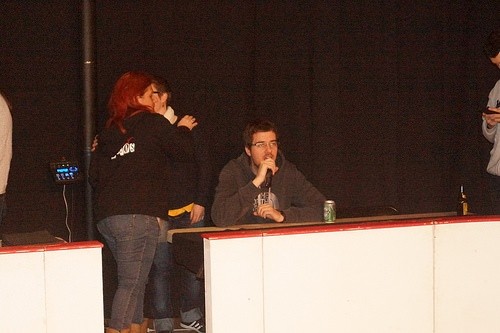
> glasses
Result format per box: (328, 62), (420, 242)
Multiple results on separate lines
(251, 142), (280, 148)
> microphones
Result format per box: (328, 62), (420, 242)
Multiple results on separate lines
(266, 168), (272, 187)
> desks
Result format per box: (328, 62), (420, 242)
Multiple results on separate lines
(167, 210), (500, 333)
(0, 237), (104, 333)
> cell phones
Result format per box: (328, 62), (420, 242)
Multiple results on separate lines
(478, 108), (499, 114)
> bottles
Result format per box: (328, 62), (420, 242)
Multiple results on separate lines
(456, 184), (468, 216)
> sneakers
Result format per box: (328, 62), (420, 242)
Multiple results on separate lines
(179, 319), (205, 333)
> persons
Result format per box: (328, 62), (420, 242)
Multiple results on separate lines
(88, 70), (213, 333)
(0, 91), (13, 248)
(482, 30), (500, 214)
(211, 117), (328, 228)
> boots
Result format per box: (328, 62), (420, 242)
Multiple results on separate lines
(130, 320), (148, 333)
(105, 327), (130, 333)
